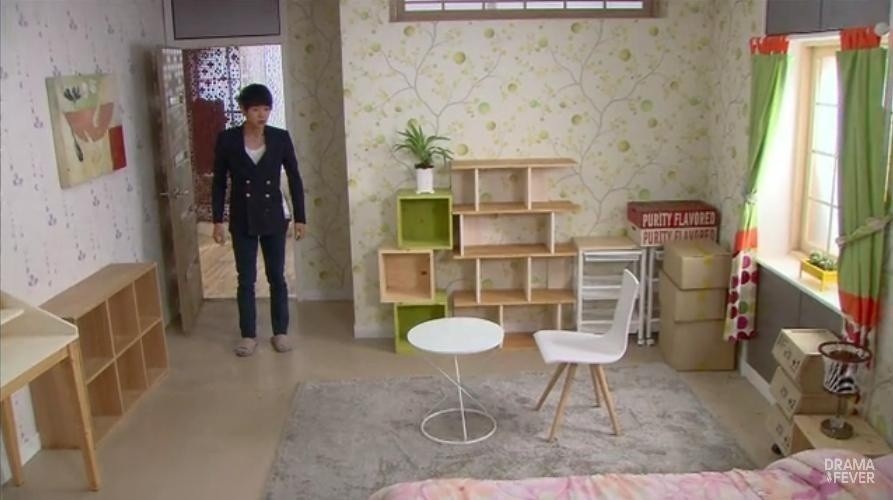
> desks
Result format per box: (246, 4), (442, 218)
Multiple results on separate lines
(407, 317), (506, 445)
(0, 288), (100, 499)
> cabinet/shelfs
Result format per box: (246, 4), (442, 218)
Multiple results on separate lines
(36, 260), (172, 448)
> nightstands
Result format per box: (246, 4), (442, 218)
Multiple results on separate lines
(791, 413), (890, 464)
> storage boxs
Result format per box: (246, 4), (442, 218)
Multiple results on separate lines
(653, 270), (726, 323)
(624, 200), (718, 246)
(663, 237), (730, 290)
(656, 309), (736, 372)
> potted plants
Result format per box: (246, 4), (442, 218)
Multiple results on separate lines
(797, 250), (838, 291)
(390, 117), (453, 194)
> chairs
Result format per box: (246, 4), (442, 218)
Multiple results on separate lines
(533, 268), (639, 442)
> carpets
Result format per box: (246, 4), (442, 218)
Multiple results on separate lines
(263, 376), (760, 500)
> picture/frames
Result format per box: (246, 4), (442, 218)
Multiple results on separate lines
(389, 0), (662, 23)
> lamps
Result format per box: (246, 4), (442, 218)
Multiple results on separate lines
(819, 340), (872, 440)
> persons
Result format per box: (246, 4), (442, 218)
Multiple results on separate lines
(211, 82), (306, 357)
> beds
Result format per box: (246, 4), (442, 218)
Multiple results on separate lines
(366, 448), (893, 499)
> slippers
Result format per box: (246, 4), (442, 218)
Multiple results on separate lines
(235, 337), (257, 356)
(271, 334), (294, 353)
